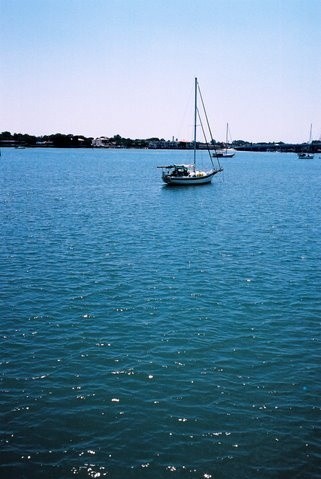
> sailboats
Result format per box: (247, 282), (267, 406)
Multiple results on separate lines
(297, 123), (315, 160)
(211, 122), (236, 158)
(156, 77), (225, 185)
